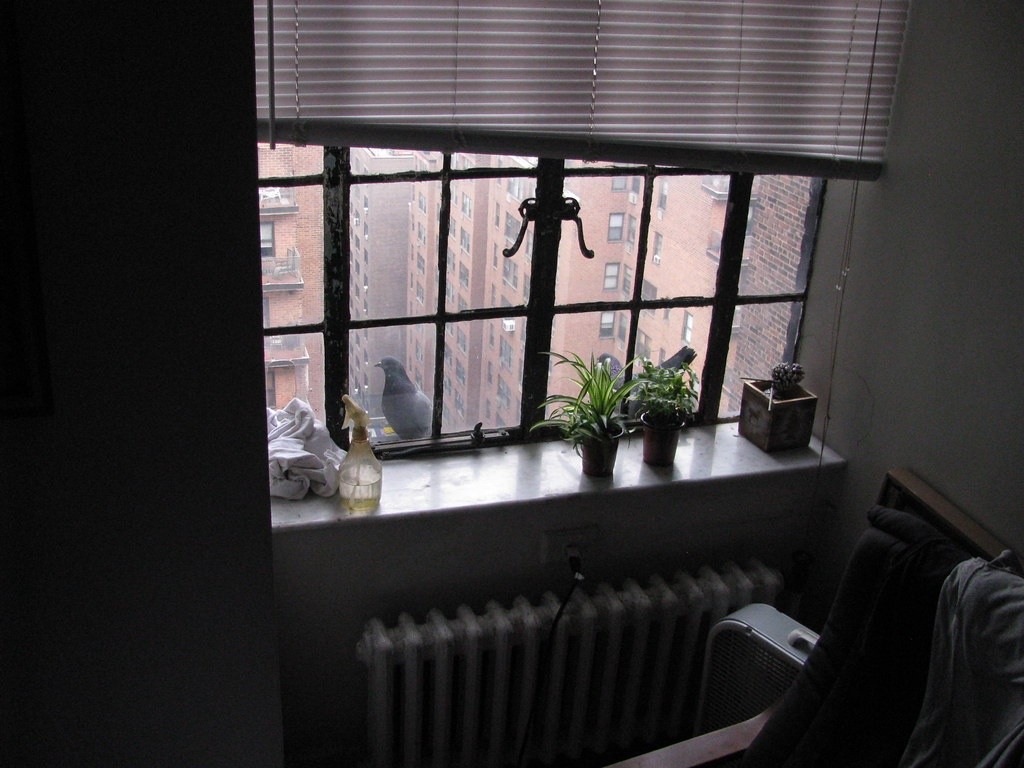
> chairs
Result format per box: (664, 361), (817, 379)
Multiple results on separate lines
(603, 505), (1023, 768)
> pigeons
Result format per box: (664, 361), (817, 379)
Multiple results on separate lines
(373, 356), (434, 441)
(596, 345), (698, 403)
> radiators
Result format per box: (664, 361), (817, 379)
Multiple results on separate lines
(355, 556), (788, 768)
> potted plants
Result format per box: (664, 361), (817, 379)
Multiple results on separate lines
(738, 361), (817, 454)
(528, 348), (656, 477)
(629, 354), (700, 467)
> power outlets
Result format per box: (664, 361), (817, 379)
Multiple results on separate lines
(541, 525), (601, 563)
